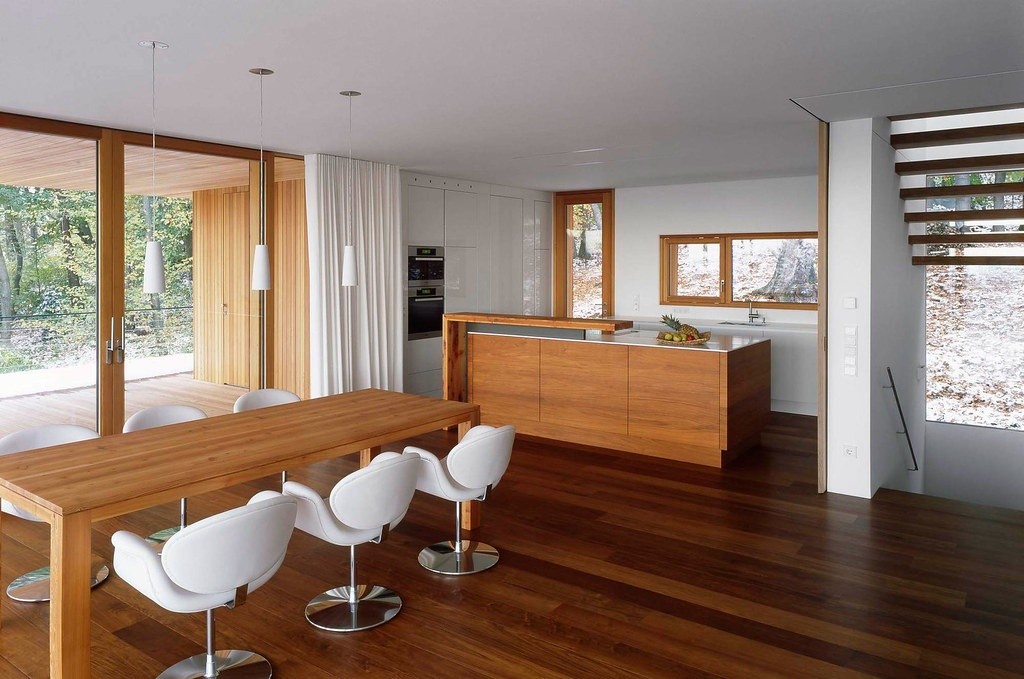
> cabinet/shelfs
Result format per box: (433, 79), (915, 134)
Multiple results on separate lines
(633, 322), (817, 417)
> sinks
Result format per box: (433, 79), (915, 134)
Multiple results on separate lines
(717, 321), (770, 326)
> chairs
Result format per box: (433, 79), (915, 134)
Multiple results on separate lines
(111, 490), (297, 679)
(0, 424), (110, 602)
(234, 389), (300, 487)
(124, 404), (208, 557)
(281, 452), (420, 631)
(402, 424), (516, 576)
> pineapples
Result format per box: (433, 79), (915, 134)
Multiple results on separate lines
(660, 314), (699, 336)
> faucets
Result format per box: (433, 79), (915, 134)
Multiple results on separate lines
(744, 298), (759, 323)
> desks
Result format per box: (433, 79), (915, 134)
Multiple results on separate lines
(0, 388), (480, 679)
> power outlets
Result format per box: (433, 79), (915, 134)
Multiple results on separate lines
(632, 295), (639, 312)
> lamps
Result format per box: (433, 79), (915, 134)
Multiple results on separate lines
(249, 68), (273, 291)
(137, 40), (169, 294)
(339, 91), (363, 287)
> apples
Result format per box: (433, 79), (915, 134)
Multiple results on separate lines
(664, 332), (693, 341)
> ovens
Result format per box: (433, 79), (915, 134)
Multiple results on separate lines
(407, 245), (445, 341)
